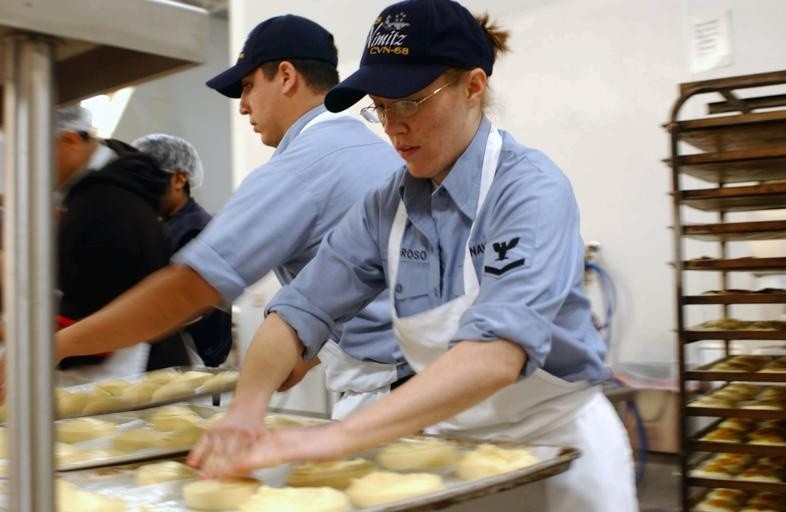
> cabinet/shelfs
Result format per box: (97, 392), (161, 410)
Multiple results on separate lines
(660, 69), (786, 512)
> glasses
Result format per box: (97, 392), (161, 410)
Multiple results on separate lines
(360, 78), (457, 123)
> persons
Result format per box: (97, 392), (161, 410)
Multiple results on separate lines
(2, 13), (417, 420)
(51, 105), (234, 369)
(188, 0), (640, 511)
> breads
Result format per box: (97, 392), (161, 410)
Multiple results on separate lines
(0, 370), (538, 511)
(688, 317), (785, 512)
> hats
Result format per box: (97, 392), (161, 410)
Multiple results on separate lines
(206, 14), (338, 98)
(324, 0), (496, 114)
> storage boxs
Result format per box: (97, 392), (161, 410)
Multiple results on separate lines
(618, 362), (704, 454)
